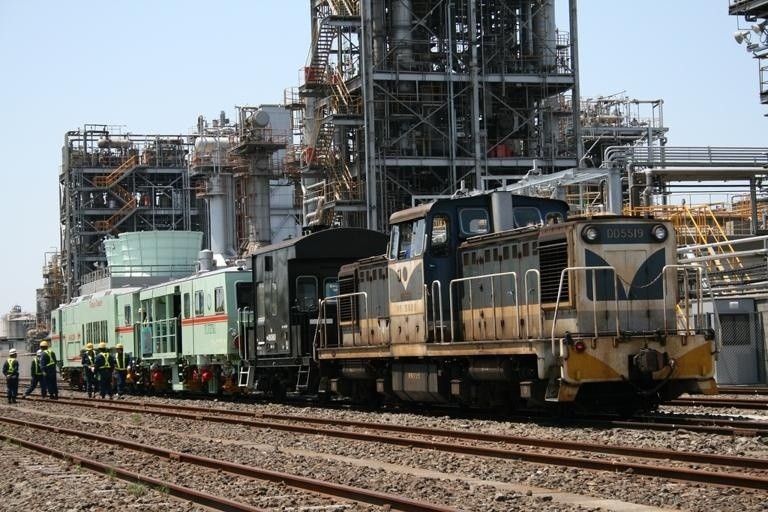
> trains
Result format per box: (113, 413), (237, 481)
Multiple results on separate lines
(50, 188), (722, 414)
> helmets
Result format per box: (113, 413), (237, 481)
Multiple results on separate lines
(86, 342), (124, 351)
(37, 341), (49, 356)
(9, 348), (18, 356)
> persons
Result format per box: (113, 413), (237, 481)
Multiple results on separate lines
(22, 341), (59, 399)
(138, 307), (152, 327)
(81, 342), (129, 400)
(2, 348), (19, 403)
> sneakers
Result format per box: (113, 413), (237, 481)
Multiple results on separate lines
(43, 393), (58, 399)
(8, 398), (16, 403)
(88, 392), (125, 400)
